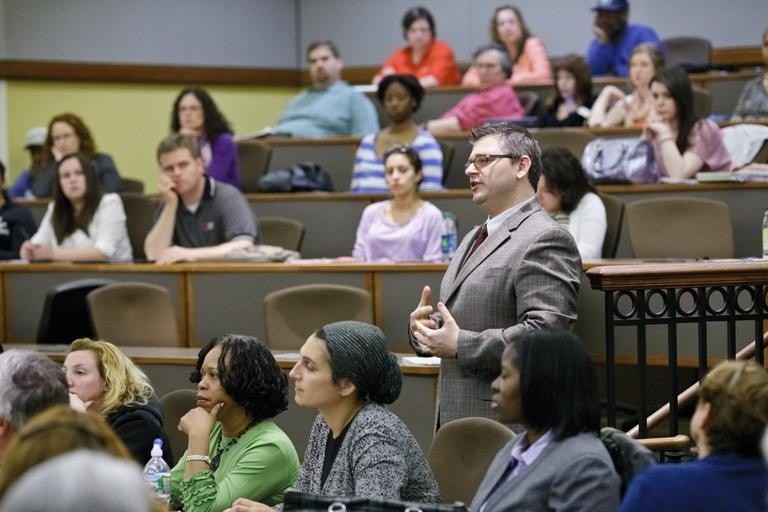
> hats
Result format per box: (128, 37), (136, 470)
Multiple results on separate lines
(22, 127), (48, 149)
(590, 0), (630, 12)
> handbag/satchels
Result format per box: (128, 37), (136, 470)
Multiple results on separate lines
(580, 137), (659, 186)
(257, 160), (335, 193)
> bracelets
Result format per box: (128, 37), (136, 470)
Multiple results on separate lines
(182, 455), (213, 466)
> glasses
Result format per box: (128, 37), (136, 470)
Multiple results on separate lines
(464, 154), (518, 169)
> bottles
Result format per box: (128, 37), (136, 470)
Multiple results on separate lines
(137, 442), (173, 508)
(440, 211), (458, 264)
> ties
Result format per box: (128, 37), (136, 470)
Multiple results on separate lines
(457, 223), (488, 275)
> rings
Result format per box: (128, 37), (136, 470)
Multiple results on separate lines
(177, 424), (184, 431)
(425, 346), (430, 353)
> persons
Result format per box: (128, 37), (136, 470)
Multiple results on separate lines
(158, 332), (302, 511)
(534, 147), (607, 265)
(468, 327), (624, 511)
(585, 1), (669, 77)
(141, 134), (261, 266)
(586, 41), (667, 129)
(532, 50), (599, 130)
(169, 87), (241, 190)
(1, 347), (70, 458)
(272, 38), (382, 138)
(1, 397), (133, 501)
(370, 4), (463, 88)
(220, 320), (443, 511)
(421, 43), (524, 132)
(0, 114), (123, 261)
(351, 144), (443, 265)
(406, 118), (585, 443)
(17, 151), (136, 264)
(348, 74), (442, 194)
(730, 28), (768, 121)
(62, 336), (175, 470)
(617, 360), (767, 512)
(460, 5), (556, 86)
(638, 65), (735, 179)
(1, 444), (171, 511)
